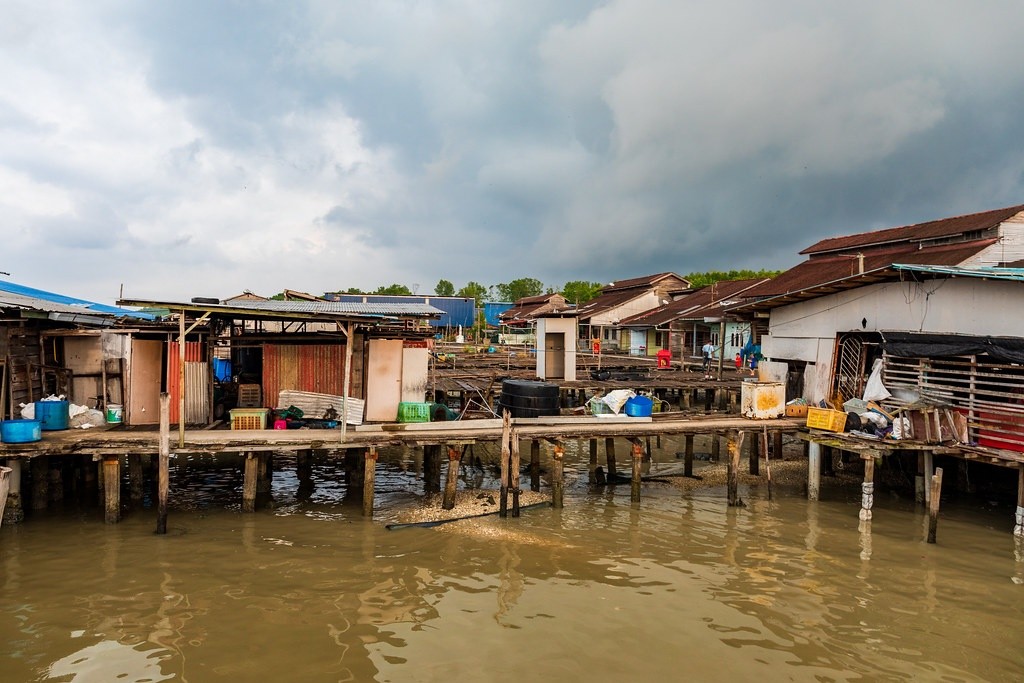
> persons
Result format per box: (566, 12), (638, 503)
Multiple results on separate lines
(734, 352), (743, 375)
(701, 339), (715, 377)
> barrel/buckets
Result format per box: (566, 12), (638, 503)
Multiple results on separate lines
(33, 399), (72, 431)
(105, 404), (124, 423)
(2, 419), (43, 444)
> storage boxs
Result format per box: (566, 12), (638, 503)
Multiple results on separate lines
(35, 401), (69, 429)
(396, 402), (430, 422)
(806, 406), (849, 432)
(625, 396), (653, 416)
(229, 408), (269, 429)
(785, 405), (808, 417)
(843, 398), (868, 414)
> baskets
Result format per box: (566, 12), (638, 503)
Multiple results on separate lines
(805, 407), (848, 432)
(239, 384), (261, 408)
(230, 408), (269, 430)
(396, 403), (431, 423)
(592, 400), (613, 415)
